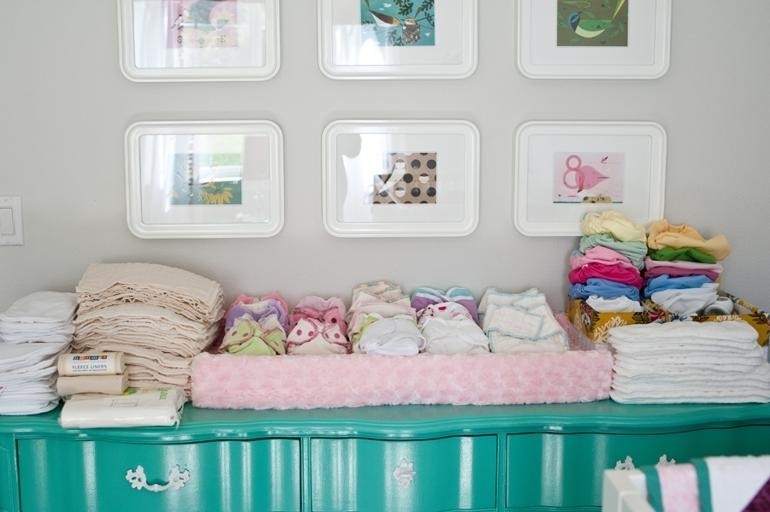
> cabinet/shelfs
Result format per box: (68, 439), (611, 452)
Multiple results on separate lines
(1, 407), (766, 512)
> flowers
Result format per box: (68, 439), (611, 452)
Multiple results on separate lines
(195, 175), (232, 203)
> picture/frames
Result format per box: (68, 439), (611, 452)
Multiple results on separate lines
(122, 117), (287, 239)
(513, 0), (674, 80)
(321, 114), (483, 238)
(316, 0), (479, 80)
(512, 121), (667, 238)
(117, 1), (284, 82)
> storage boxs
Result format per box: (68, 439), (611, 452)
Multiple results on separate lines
(194, 306), (615, 405)
(600, 455), (768, 511)
(566, 285), (768, 353)
